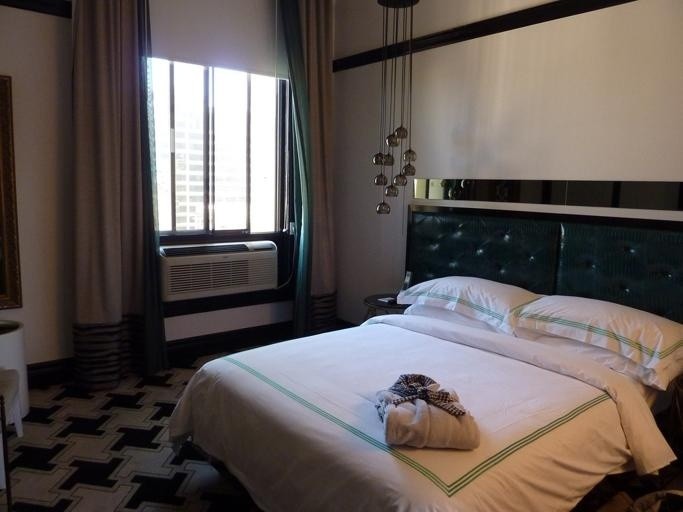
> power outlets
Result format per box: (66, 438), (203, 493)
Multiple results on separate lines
(288, 221), (295, 236)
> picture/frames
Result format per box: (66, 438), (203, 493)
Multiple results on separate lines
(0, 74), (22, 311)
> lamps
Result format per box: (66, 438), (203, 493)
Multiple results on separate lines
(371, 0), (417, 217)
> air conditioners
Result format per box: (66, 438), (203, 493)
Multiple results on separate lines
(158, 239), (278, 303)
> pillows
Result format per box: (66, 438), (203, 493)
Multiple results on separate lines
(395, 275), (682, 391)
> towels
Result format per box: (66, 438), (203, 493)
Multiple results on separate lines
(374, 388), (481, 451)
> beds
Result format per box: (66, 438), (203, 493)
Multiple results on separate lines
(166, 180), (682, 511)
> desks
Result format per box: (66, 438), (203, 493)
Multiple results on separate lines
(363, 293), (411, 322)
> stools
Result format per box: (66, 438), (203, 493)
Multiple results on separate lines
(0, 367), (24, 490)
